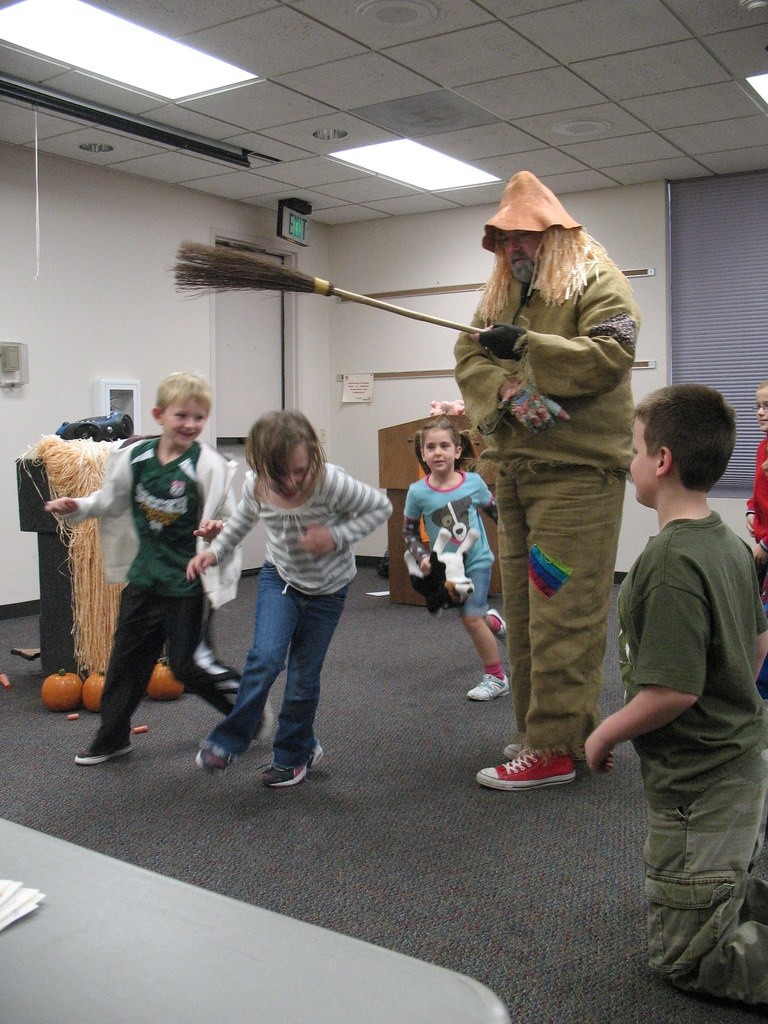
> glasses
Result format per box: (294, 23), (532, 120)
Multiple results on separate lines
(752, 401), (768, 413)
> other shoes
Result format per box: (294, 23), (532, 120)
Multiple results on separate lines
(254, 702), (273, 741)
(75, 740), (133, 764)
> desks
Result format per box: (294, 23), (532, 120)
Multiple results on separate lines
(0, 819), (510, 1024)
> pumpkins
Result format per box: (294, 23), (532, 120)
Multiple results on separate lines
(41, 668), (83, 712)
(82, 670), (107, 712)
(146, 660), (184, 701)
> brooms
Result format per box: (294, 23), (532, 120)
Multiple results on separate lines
(166, 239), (485, 333)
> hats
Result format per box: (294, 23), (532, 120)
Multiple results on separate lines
(482, 172), (582, 253)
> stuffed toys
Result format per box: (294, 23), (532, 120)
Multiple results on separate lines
(430, 396), (466, 415)
(401, 527), (480, 618)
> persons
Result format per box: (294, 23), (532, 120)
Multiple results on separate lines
(585, 383), (768, 1005)
(186, 408), (394, 787)
(402, 419), (509, 702)
(45, 371), (272, 765)
(453, 170), (638, 789)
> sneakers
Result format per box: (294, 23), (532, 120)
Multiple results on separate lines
(196, 739), (258, 773)
(487, 608), (509, 648)
(476, 750), (576, 790)
(256, 743), (324, 786)
(467, 673), (509, 701)
(504, 743), (587, 761)
